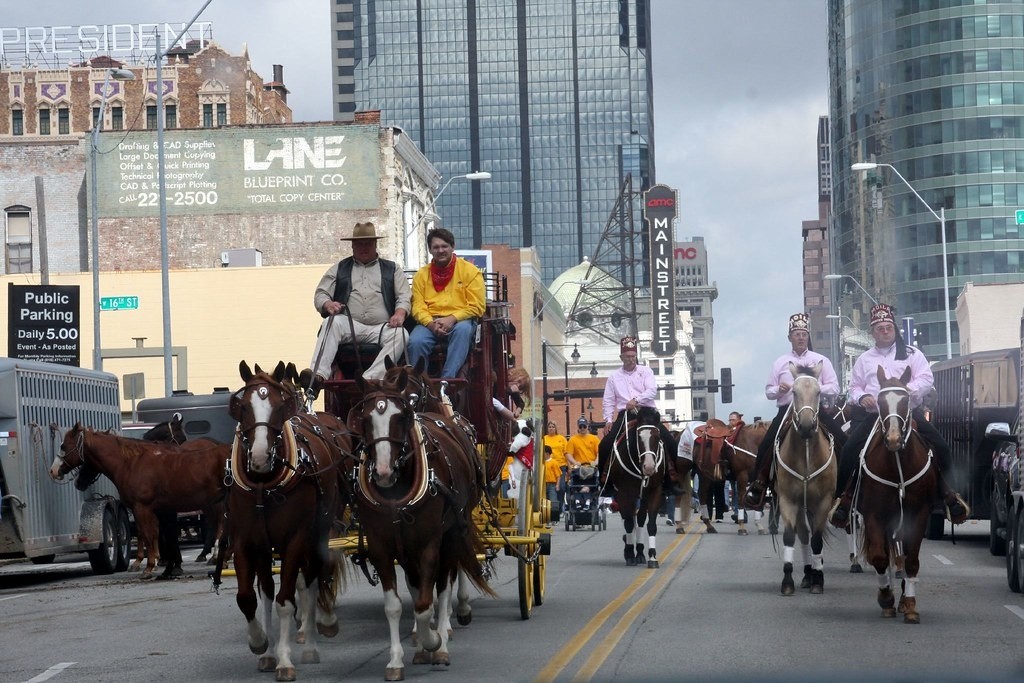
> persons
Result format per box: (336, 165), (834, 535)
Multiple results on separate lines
(828, 303), (970, 528)
(490, 367), (532, 418)
(743, 313), (860, 535)
(407, 228), (486, 380)
(300, 221), (411, 400)
(660, 412), (747, 526)
(502, 418), (602, 529)
(598, 337), (686, 497)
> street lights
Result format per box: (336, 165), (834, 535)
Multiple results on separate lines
(88, 68), (137, 372)
(542, 338), (581, 440)
(564, 360), (599, 440)
(851, 161), (953, 360)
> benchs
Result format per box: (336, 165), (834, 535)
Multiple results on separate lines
(328, 318), (517, 352)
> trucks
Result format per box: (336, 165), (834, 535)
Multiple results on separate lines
(923, 307), (1024, 593)
(120, 387), (249, 559)
(0, 356), (134, 576)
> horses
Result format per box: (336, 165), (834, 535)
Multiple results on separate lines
(847, 365), (938, 624)
(671, 421), (773, 536)
(773, 360), (838, 598)
(350, 354), (501, 682)
(229, 360), (352, 682)
(49, 413), (232, 582)
(609, 408), (668, 569)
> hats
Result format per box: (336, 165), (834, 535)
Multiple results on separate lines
(789, 314), (810, 333)
(577, 418), (588, 425)
(620, 337), (638, 353)
(870, 303), (894, 325)
(340, 222), (387, 241)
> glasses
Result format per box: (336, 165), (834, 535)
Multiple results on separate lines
(579, 425), (589, 428)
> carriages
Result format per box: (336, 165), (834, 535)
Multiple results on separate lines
(221, 268), (553, 682)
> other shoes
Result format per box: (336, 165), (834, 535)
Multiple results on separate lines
(746, 490), (767, 512)
(947, 500), (966, 525)
(731, 514), (738, 524)
(716, 519), (724, 523)
(553, 520), (560, 526)
(666, 519), (674, 526)
(832, 496), (852, 521)
(673, 482), (687, 496)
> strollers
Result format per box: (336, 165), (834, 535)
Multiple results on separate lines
(563, 466), (607, 533)
